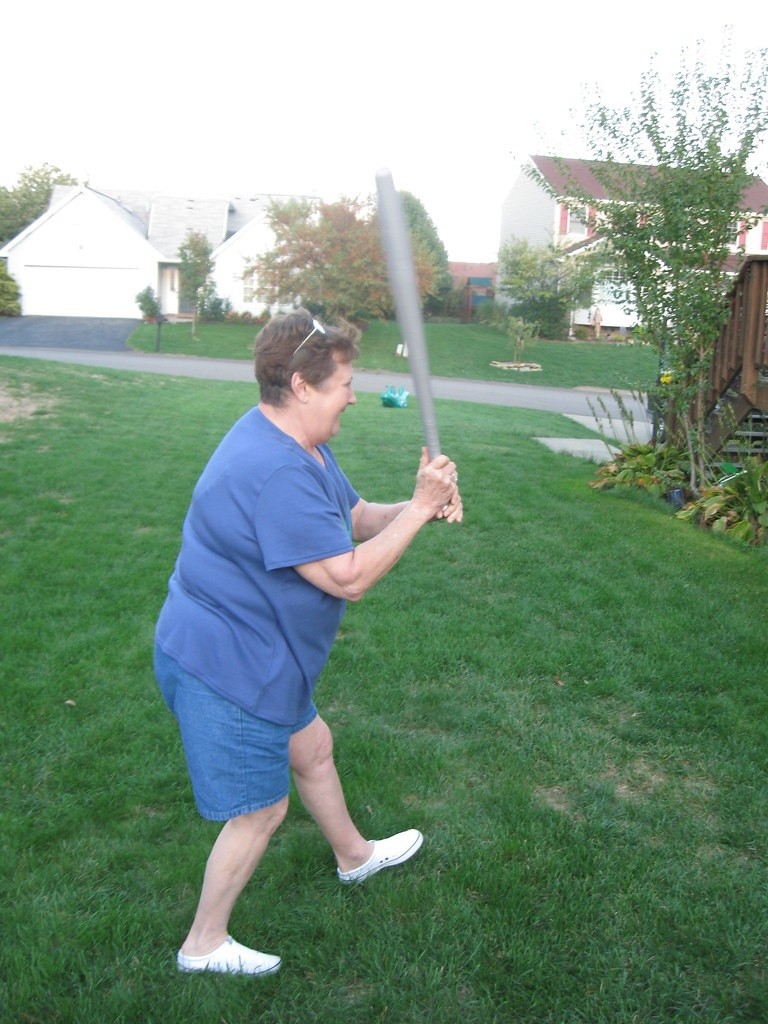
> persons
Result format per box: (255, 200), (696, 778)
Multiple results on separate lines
(154, 310), (464, 975)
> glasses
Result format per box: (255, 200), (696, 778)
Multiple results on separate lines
(292, 319), (326, 354)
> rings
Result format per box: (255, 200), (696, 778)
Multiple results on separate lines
(451, 473), (457, 482)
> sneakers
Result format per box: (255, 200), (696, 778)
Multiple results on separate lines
(337, 829), (424, 887)
(177, 936), (283, 976)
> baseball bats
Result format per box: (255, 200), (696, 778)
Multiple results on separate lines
(376, 167), (450, 513)
